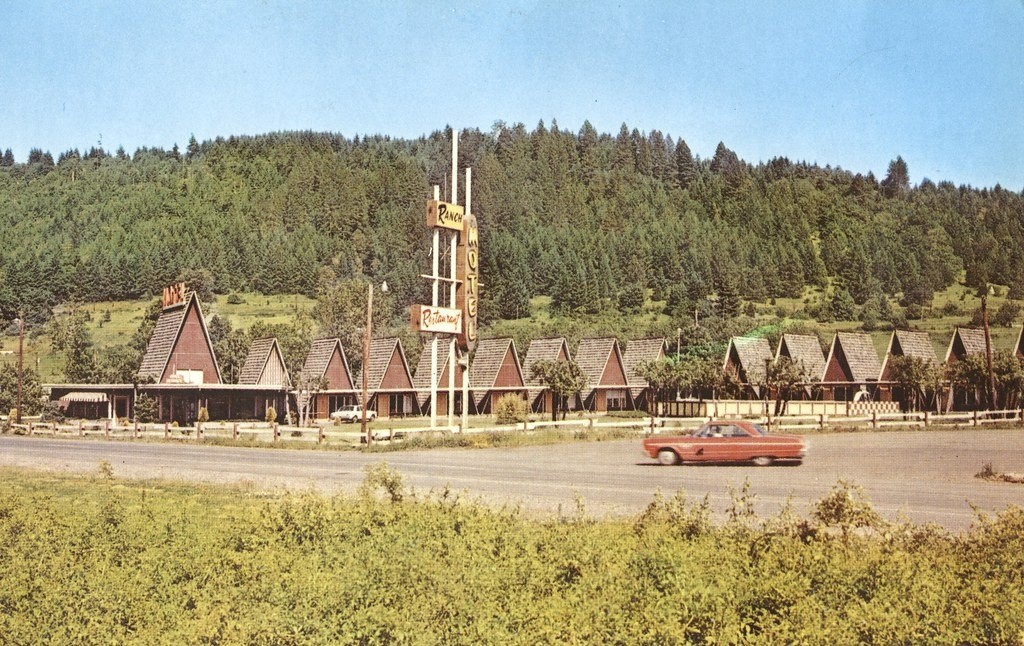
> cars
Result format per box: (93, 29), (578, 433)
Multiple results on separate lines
(330, 404), (378, 424)
(643, 416), (807, 469)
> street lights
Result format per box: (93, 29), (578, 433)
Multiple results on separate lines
(13, 310), (24, 427)
(360, 279), (390, 445)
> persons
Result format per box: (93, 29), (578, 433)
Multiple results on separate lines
(712, 427), (723, 437)
